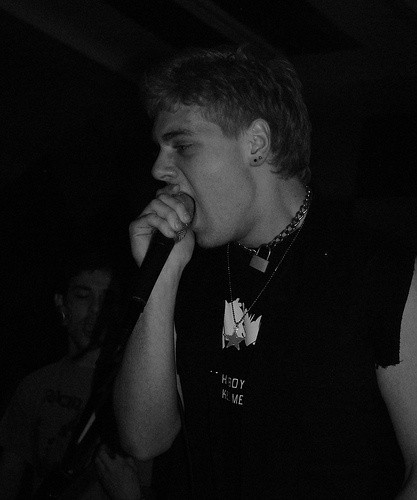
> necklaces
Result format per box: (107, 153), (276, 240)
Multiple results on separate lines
(223, 186), (315, 350)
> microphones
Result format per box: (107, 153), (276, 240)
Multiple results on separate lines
(131, 192), (195, 304)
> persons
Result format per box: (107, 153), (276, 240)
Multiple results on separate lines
(0, 242), (152, 500)
(113, 43), (417, 500)
